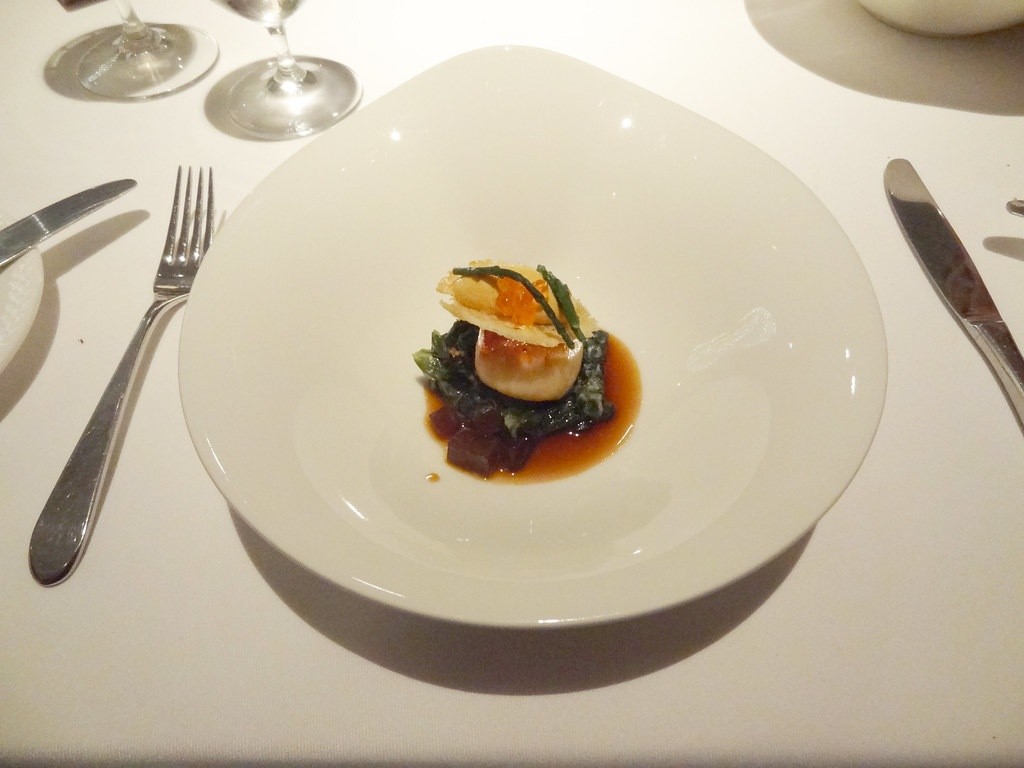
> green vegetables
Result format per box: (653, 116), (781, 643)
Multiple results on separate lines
(411, 264), (616, 443)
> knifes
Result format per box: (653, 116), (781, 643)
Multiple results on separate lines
(0, 179), (137, 269)
(885, 158), (1024, 423)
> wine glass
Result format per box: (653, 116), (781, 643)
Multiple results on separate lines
(214, 0), (362, 140)
(41, 0), (222, 102)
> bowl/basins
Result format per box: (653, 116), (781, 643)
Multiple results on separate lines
(858, 0), (1024, 38)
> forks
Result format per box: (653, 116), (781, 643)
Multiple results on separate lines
(28, 164), (214, 587)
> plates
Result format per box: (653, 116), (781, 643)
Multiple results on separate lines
(174, 43), (889, 629)
(0, 223), (44, 375)
